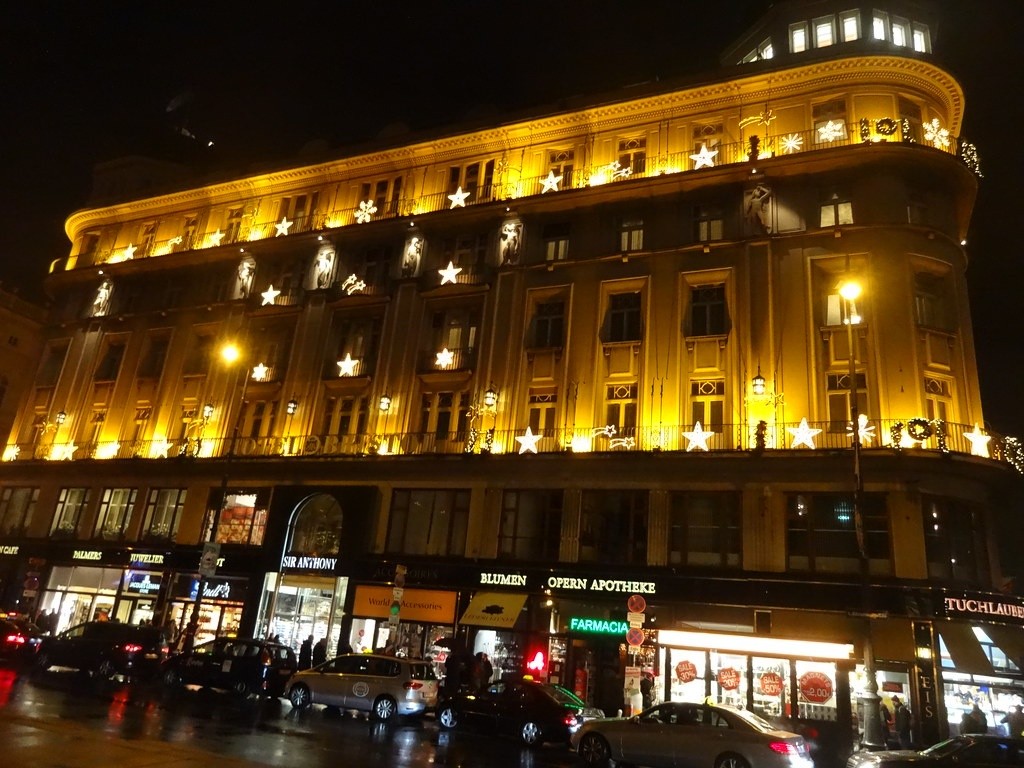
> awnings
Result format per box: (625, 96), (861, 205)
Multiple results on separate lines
(459, 590), (528, 629)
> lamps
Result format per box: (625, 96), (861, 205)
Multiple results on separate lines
(287, 392), (297, 414)
(922, 117), (950, 149)
(484, 382), (498, 408)
(54, 405), (67, 424)
(438, 261), (463, 286)
(515, 426), (544, 455)
(274, 215), (294, 236)
(123, 243), (138, 261)
(209, 228), (226, 248)
(786, 418), (823, 450)
(688, 143), (719, 169)
(341, 273), (366, 296)
(353, 200), (378, 225)
(435, 348), (454, 368)
(5, 436), (173, 461)
(251, 363), (269, 381)
(964, 423), (992, 458)
(681, 420), (716, 452)
(592, 424), (636, 452)
(448, 186), (471, 210)
(336, 353), (359, 377)
(596, 159), (634, 181)
(780, 132), (805, 155)
(847, 418), (877, 446)
(752, 366), (765, 395)
(202, 396), (213, 417)
(260, 284), (281, 306)
(379, 388), (391, 411)
(157, 235), (184, 254)
(539, 170), (563, 194)
(817, 120), (843, 142)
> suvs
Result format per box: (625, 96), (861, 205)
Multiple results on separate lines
(31, 620), (174, 684)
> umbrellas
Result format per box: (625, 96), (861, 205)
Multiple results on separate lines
(433, 637), (466, 652)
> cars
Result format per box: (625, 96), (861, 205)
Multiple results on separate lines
(569, 700), (814, 768)
(157, 636), (297, 701)
(436, 678), (607, 749)
(0, 617), (42, 657)
(846, 732), (1024, 768)
(282, 652), (440, 722)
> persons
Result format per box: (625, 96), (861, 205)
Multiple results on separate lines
(35, 608), (58, 635)
(336, 639), (353, 656)
(383, 639), (395, 656)
(361, 646), (373, 654)
(891, 695), (910, 750)
(959, 705), (987, 735)
(164, 619), (179, 656)
(641, 673), (656, 710)
(1000, 705), (1024, 737)
(880, 704), (892, 744)
(313, 638), (327, 666)
(139, 618), (151, 626)
(446, 651), (492, 694)
(268, 633), (279, 642)
(298, 635), (314, 665)
